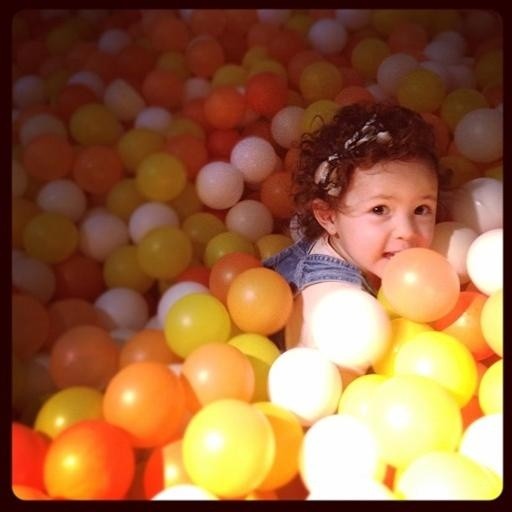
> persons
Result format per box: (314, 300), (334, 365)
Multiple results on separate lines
(263, 102), (454, 354)
